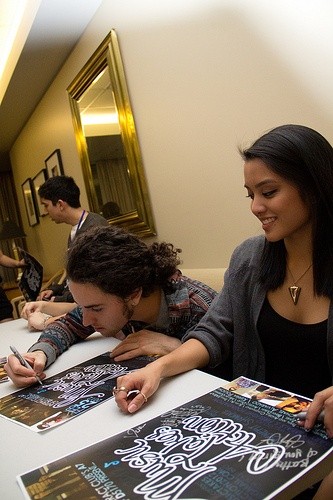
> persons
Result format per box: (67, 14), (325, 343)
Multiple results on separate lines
(0, 177), (127, 342)
(3, 226), (233, 387)
(113, 124), (333, 439)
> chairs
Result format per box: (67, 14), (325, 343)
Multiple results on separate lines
(10, 268), (66, 320)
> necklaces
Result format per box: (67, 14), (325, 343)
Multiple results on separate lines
(286, 263), (313, 305)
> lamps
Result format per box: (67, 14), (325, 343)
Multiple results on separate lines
(0, 217), (27, 282)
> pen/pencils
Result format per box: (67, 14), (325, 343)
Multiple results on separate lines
(295, 413), (326, 421)
(9, 344), (43, 386)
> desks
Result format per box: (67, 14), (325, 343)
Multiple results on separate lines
(0, 318), (333, 500)
(2, 276), (49, 300)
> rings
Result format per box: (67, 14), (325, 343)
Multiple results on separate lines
(113, 386), (129, 393)
(139, 392), (147, 402)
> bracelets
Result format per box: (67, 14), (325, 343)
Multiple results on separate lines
(42, 315), (52, 328)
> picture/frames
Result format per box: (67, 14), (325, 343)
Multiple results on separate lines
(45, 149), (64, 179)
(31, 169), (50, 217)
(21, 178), (40, 227)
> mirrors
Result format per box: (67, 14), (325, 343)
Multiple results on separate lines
(66, 29), (158, 240)
